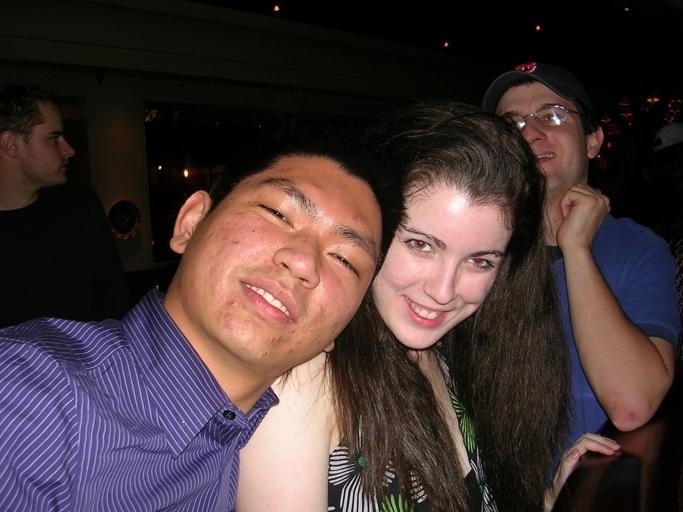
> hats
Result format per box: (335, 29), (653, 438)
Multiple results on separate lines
(483, 61), (592, 129)
(652, 122), (683, 153)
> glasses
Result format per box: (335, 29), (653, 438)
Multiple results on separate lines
(502, 103), (581, 132)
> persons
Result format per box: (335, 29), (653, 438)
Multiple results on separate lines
(0, 87), (128, 330)
(487, 54), (682, 485)
(233, 98), (623, 511)
(0, 116), (409, 512)
